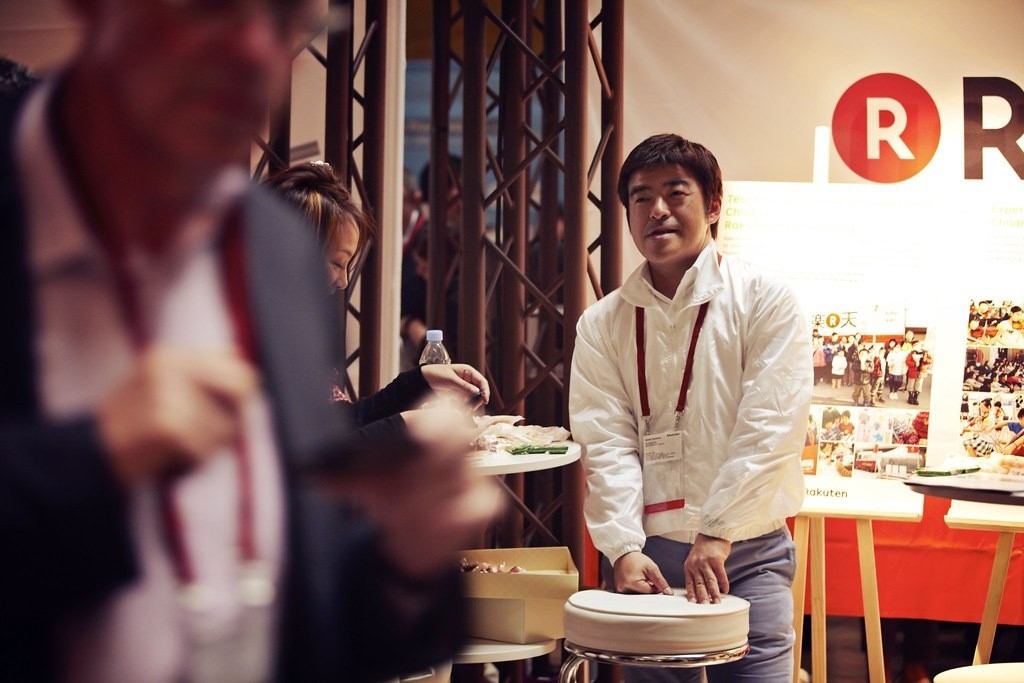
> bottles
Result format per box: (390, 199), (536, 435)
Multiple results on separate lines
(418, 329), (451, 365)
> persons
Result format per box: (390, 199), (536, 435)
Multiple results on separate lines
(804, 408), (855, 461)
(960, 392), (1024, 459)
(266, 163), (487, 430)
(968, 300), (1024, 347)
(397, 154), (561, 418)
(0, 1), (510, 682)
(568, 134), (813, 683)
(810, 326), (931, 407)
(963, 353), (1024, 394)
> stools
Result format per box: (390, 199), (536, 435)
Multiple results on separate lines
(557, 584), (750, 683)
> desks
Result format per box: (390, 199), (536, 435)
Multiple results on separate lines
(463, 439), (583, 475)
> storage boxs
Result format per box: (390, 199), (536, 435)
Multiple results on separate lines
(460, 596), (574, 645)
(457, 547), (579, 602)
